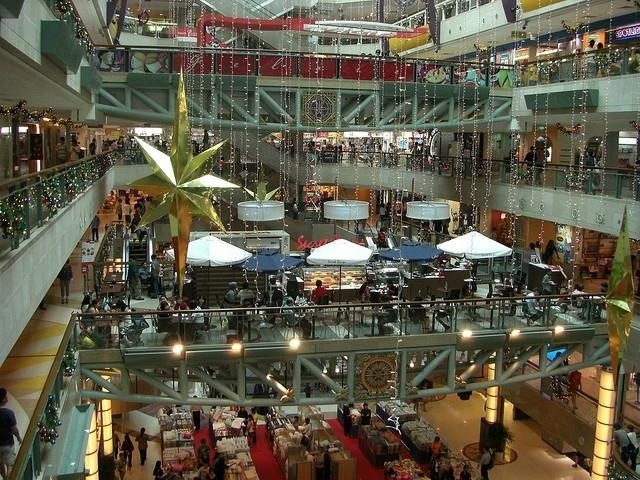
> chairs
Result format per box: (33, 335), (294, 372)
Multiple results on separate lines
(79, 249), (622, 343)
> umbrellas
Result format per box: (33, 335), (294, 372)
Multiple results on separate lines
(306, 237), (374, 309)
(434, 230), (515, 299)
(377, 238), (445, 304)
(164, 233), (255, 325)
(231, 247), (304, 293)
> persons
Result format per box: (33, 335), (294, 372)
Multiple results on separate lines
(570, 449), (580, 468)
(112, 382), (372, 480)
(550, 351), (582, 411)
(55, 135), (168, 164)
(0, 386), (25, 480)
(525, 236), (607, 328)
(520, 145), (537, 171)
(39, 190), (206, 346)
(220, 276), (457, 343)
(585, 149), (595, 172)
(535, 146), (546, 185)
(270, 134), (434, 171)
(429, 434), (497, 480)
(612, 423), (630, 466)
(583, 39), (597, 79)
(625, 423), (639, 473)
(290, 197), (299, 220)
(595, 42), (607, 78)
(377, 196), (451, 236)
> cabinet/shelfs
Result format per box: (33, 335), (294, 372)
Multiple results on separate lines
(156, 411), (474, 480)
(581, 228), (619, 259)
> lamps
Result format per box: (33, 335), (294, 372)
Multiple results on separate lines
(485, 362), (507, 427)
(84, 406), (101, 480)
(98, 373), (117, 456)
(590, 371), (619, 479)
